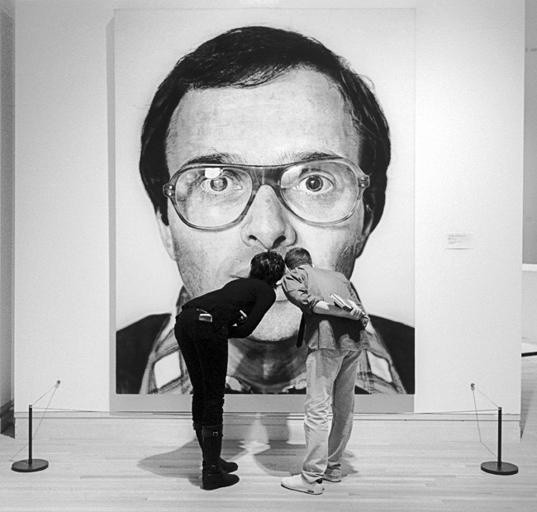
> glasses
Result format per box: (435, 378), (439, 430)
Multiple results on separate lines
(162, 157), (370, 233)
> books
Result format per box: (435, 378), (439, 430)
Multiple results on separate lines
(329, 293), (369, 320)
(333, 293), (353, 310)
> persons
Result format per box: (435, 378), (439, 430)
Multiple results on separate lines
(274, 244), (371, 498)
(170, 249), (289, 494)
(112, 23), (417, 398)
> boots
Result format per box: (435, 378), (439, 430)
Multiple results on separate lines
(200, 423), (239, 490)
(193, 421), (238, 474)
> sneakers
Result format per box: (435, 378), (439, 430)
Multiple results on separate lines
(280, 474), (324, 496)
(323, 466), (342, 483)
